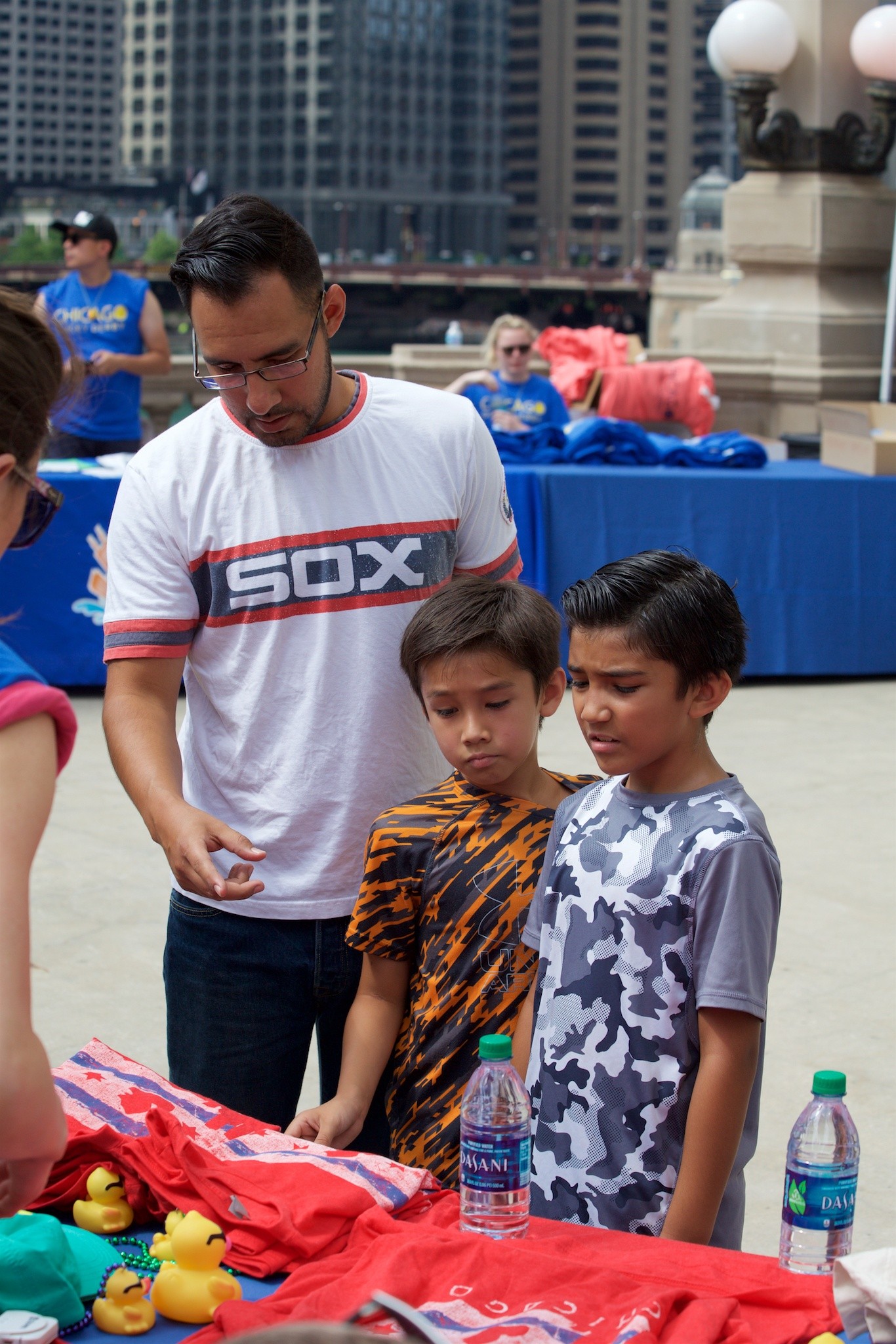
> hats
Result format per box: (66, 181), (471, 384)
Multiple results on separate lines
(52, 210), (117, 243)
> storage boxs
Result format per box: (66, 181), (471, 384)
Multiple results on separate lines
(818, 400), (896, 475)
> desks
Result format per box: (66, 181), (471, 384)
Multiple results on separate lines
(0, 460), (896, 684)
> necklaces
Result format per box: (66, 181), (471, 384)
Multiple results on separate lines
(74, 271), (113, 315)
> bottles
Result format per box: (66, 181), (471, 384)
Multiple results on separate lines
(778, 1070), (861, 1274)
(460, 1034), (532, 1239)
(446, 320), (462, 345)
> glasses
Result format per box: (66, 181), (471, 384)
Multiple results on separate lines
(496, 345), (532, 355)
(61, 231), (99, 245)
(8, 465), (63, 552)
(194, 287), (326, 391)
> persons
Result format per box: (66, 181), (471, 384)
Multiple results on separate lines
(29, 211), (171, 458)
(0, 298), (71, 1223)
(102, 198), (524, 1158)
(522, 548), (783, 1254)
(442, 313), (567, 430)
(282, 576), (605, 1193)
